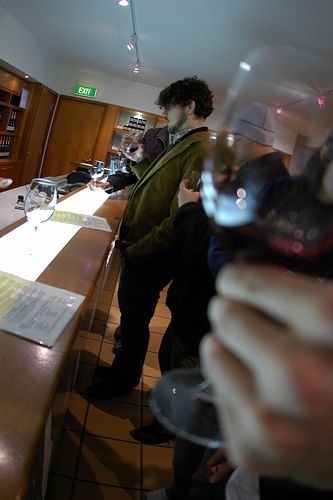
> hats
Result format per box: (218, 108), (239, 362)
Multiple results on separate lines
(231, 102), (276, 146)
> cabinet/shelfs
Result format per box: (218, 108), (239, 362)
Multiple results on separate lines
(0, 65), (36, 194)
(106, 108), (160, 157)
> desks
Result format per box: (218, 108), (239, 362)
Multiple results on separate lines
(0, 169), (132, 500)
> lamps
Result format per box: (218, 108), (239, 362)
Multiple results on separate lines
(133, 60), (140, 74)
(126, 33), (137, 51)
(119, 0), (130, 7)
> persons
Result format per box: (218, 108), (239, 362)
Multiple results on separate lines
(128, 102), (291, 445)
(86, 121), (174, 337)
(89, 74), (216, 388)
(198, 256), (332, 498)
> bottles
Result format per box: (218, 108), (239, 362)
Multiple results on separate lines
(0, 136), (11, 159)
(7, 111), (17, 132)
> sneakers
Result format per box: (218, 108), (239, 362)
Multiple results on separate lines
(86, 380), (133, 397)
(93, 365), (109, 379)
(130, 419), (174, 443)
(145, 488), (169, 500)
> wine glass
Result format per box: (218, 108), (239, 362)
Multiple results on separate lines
(114, 131), (141, 174)
(90, 159), (104, 192)
(19, 178), (58, 256)
(149, 45), (332, 449)
